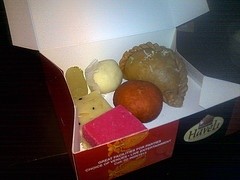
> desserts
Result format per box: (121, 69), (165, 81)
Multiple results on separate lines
(66, 59), (164, 149)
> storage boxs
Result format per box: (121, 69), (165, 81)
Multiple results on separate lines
(3, 0), (239, 180)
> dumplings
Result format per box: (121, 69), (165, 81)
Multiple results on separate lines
(119, 41), (189, 108)
